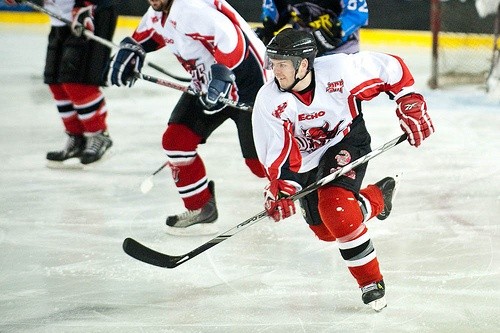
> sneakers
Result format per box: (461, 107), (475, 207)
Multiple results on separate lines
(165, 180), (219, 236)
(80, 131), (113, 169)
(45, 131), (84, 168)
(376, 176), (398, 220)
(361, 280), (388, 313)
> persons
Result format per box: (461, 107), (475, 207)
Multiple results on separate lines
(252, 28), (435, 311)
(253, 0), (368, 57)
(103, 0), (274, 235)
(2, 0), (119, 168)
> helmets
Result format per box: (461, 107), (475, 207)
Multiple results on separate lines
(266, 28), (317, 72)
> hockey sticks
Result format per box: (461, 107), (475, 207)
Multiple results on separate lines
(14, 0), (191, 81)
(140, 161), (169, 194)
(122, 130), (412, 269)
(135, 71), (255, 114)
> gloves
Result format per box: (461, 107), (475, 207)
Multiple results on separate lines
(104, 37), (144, 87)
(309, 26), (342, 55)
(71, 1), (95, 39)
(263, 179), (297, 223)
(396, 93), (435, 147)
(196, 64), (236, 114)
(250, 16), (280, 47)
(4, 0), (26, 6)
